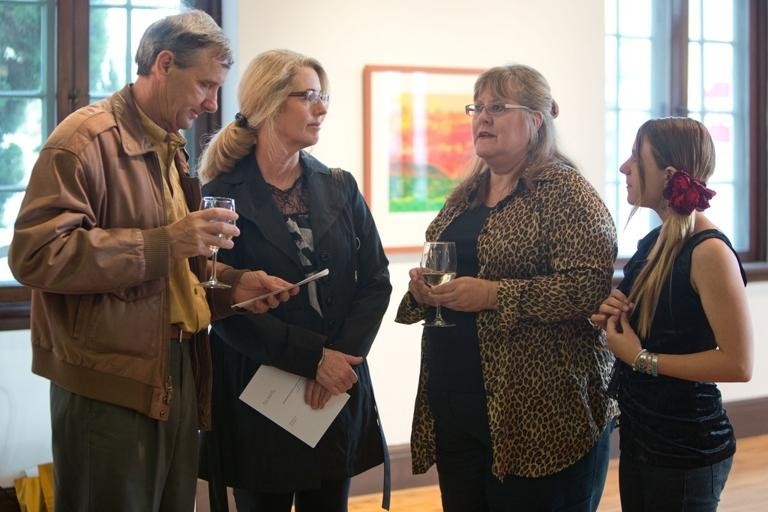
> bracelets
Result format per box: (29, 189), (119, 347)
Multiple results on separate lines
(631, 348), (660, 377)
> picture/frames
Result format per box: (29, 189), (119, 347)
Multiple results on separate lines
(362, 64), (490, 253)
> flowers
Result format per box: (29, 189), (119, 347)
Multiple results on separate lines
(662, 169), (715, 217)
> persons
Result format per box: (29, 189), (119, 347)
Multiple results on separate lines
(8, 9), (242, 512)
(589, 114), (754, 510)
(395, 66), (621, 510)
(196, 47), (392, 511)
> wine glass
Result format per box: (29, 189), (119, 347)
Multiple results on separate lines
(422, 241), (458, 329)
(198, 197), (234, 289)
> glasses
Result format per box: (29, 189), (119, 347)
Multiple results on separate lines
(465, 104), (535, 115)
(288, 89), (330, 104)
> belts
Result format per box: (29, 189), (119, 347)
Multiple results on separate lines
(170, 324), (193, 342)
